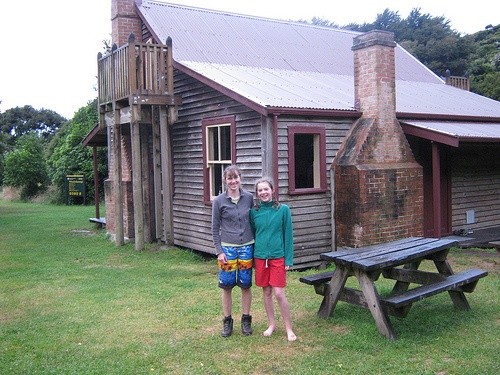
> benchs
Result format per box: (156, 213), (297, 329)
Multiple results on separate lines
(379, 268), (490, 340)
(298, 261), (417, 318)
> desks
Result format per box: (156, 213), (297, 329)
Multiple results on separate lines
(318, 236), (459, 299)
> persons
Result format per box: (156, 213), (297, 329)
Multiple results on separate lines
(249, 178), (296, 342)
(211, 165), (255, 337)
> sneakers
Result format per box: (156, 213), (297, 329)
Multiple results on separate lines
(221, 318), (233, 337)
(241, 315), (252, 336)
